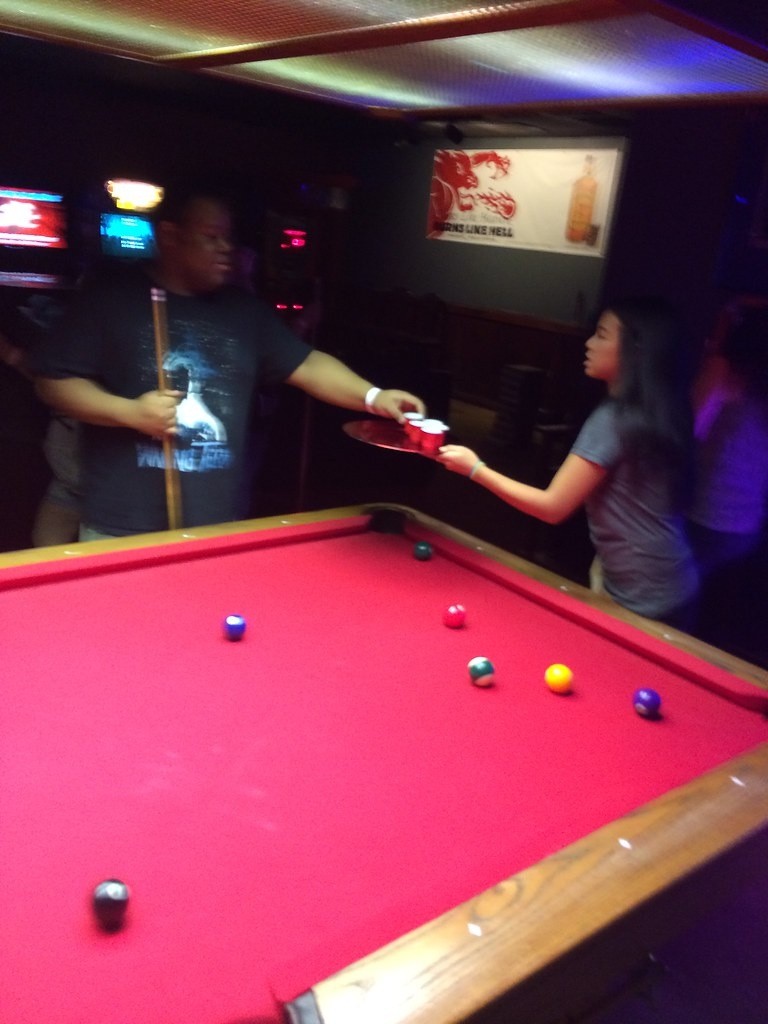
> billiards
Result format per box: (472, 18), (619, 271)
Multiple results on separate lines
(90, 878), (130, 920)
(444, 604), (467, 628)
(467, 656), (495, 688)
(224, 613), (246, 638)
(632, 688), (662, 716)
(543, 662), (573, 695)
(413, 540), (432, 559)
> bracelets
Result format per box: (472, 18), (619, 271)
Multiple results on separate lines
(365, 388), (380, 414)
(468, 461), (484, 479)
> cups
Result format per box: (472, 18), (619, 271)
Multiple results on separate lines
(400, 411), (449, 453)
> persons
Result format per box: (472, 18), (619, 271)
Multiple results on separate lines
(436, 295), (701, 636)
(0, 181), (117, 546)
(33, 180), (427, 540)
(685, 298), (768, 577)
(232, 209), (319, 333)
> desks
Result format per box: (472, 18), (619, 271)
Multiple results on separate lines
(0, 502), (768, 1024)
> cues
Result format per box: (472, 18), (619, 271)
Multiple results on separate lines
(148, 287), (188, 527)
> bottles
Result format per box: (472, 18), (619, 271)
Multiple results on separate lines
(567, 154), (597, 243)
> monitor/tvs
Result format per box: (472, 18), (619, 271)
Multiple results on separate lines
(0, 187), (68, 248)
(100, 212), (156, 258)
